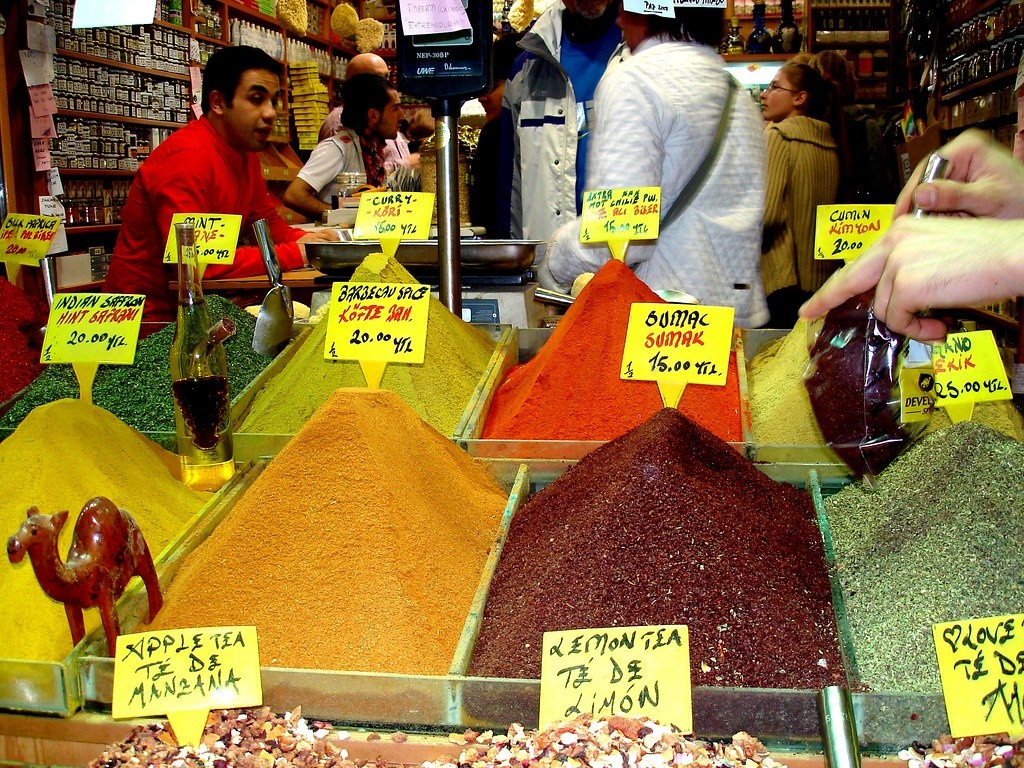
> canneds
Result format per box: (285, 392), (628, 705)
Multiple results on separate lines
(43, 0), (223, 229)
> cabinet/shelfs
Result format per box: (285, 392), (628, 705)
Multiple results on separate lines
(0, 0), (395, 292)
(719, 0), (1024, 150)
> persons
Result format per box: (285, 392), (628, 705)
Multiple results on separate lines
(278, 72), (406, 221)
(319, 50), (423, 175)
(99, 47), (338, 340)
(757, 48), (904, 330)
(467, 0), (773, 328)
(796, 124), (1023, 339)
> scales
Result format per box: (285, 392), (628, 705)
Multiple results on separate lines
(297, 1), (553, 328)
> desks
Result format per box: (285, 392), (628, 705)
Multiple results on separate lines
(168, 268), (322, 290)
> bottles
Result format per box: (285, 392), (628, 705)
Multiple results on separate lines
(725, 0), (803, 55)
(171, 221), (238, 492)
(229, 18), (349, 80)
(816, 9), (889, 30)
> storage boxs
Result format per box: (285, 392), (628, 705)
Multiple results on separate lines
(0, 320), (1024, 768)
(289, 61), (329, 150)
(55, 252), (93, 288)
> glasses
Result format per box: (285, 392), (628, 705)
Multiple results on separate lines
(763, 82), (801, 95)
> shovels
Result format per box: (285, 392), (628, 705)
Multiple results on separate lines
(251, 218), (293, 359)
